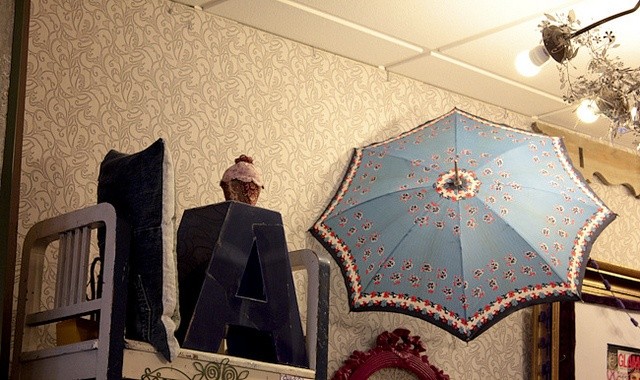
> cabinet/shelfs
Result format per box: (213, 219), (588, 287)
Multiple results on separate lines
(11, 200), (332, 380)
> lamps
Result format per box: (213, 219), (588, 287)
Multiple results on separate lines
(575, 79), (640, 125)
(516, 0), (640, 78)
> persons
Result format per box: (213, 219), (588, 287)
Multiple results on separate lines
(627, 354), (640, 380)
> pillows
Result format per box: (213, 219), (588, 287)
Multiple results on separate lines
(96, 137), (182, 363)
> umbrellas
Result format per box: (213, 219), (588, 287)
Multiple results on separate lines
(307, 105), (620, 347)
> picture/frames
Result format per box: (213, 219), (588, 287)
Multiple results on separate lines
(528, 257), (640, 379)
